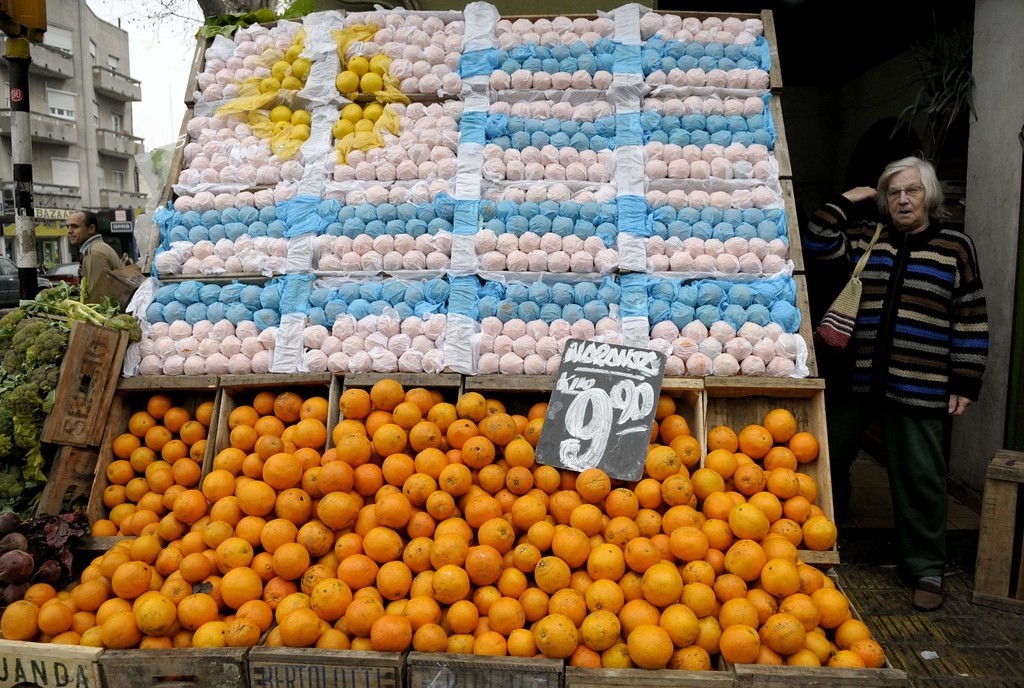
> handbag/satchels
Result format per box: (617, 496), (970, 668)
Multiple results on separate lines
(813, 223), (884, 351)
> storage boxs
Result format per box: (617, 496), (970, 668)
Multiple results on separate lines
(0, 0), (908, 688)
(968, 448), (1024, 616)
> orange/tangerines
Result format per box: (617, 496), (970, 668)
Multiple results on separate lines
(0, 376), (889, 675)
(261, 44), (394, 143)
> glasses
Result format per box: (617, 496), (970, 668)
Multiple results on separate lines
(886, 186), (923, 197)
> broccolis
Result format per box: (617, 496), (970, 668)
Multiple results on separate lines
(0, 278), (144, 514)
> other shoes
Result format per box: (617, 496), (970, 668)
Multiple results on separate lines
(914, 576), (945, 611)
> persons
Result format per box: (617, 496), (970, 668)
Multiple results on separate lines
(802, 156), (989, 613)
(66, 210), (122, 305)
(120, 253), (134, 266)
